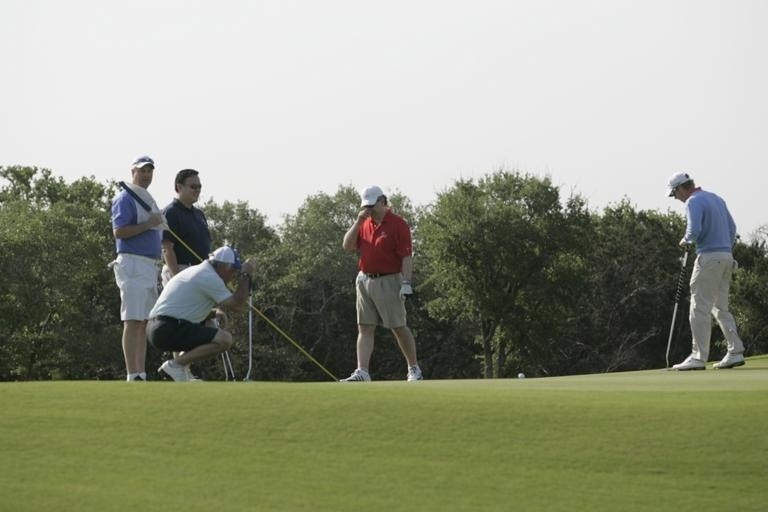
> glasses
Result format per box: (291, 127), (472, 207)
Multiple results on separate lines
(190, 184), (202, 189)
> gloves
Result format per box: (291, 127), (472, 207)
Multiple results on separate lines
(399, 281), (414, 301)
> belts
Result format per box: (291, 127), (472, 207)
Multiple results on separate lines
(367, 273), (387, 278)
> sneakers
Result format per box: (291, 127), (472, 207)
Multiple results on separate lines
(157, 360), (197, 382)
(406, 366), (424, 381)
(713, 353), (745, 370)
(339, 370), (372, 382)
(673, 355), (705, 370)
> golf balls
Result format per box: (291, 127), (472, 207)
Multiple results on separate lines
(518, 373), (525, 379)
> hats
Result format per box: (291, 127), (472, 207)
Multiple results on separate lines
(360, 185), (383, 208)
(210, 245), (242, 269)
(667, 172), (693, 196)
(131, 156), (154, 169)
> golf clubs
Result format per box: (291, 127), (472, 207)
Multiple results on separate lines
(666, 251), (690, 366)
(242, 288), (252, 381)
(224, 350), (238, 382)
(221, 353), (228, 381)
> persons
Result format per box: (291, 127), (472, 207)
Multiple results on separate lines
(340, 186), (425, 382)
(668, 173), (745, 370)
(143, 244), (255, 381)
(159, 168), (212, 381)
(105, 156), (168, 382)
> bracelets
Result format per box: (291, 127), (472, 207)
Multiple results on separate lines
(239, 272), (251, 281)
